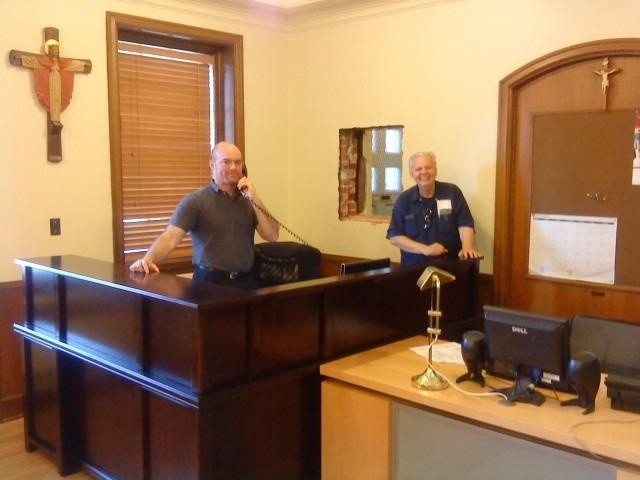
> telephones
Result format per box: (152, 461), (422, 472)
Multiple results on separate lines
(234, 162), (247, 191)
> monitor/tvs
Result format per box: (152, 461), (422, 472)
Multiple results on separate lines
(482, 303), (569, 407)
(340, 257), (390, 275)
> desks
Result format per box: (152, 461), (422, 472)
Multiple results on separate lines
(319, 331), (640, 480)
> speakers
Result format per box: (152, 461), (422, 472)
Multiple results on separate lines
(456, 330), (484, 386)
(560, 351), (600, 415)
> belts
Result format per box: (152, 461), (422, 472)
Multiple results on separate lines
(195, 264), (245, 282)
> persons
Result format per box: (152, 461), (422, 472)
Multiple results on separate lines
(130, 141), (280, 292)
(385, 150), (481, 266)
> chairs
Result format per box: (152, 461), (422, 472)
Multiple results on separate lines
(570, 314), (640, 380)
(255, 242), (321, 288)
